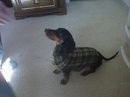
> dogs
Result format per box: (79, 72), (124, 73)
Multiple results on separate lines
(44, 28), (120, 85)
